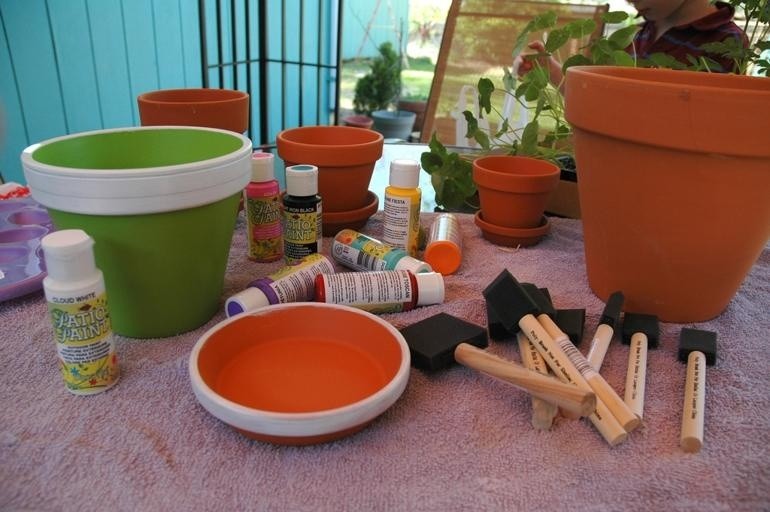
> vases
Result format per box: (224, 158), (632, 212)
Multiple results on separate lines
(344, 115), (374, 130)
(565, 65), (770, 325)
(397, 102), (428, 132)
(278, 125), (385, 236)
(21, 125), (255, 340)
(373, 109), (417, 140)
(472, 153), (561, 249)
(138, 88), (251, 211)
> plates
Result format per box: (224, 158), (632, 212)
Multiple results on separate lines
(0, 195), (60, 304)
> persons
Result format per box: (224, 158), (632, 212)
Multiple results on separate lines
(508, 2), (755, 109)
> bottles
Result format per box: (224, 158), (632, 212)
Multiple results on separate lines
(225, 152), (465, 317)
(40, 227), (122, 397)
